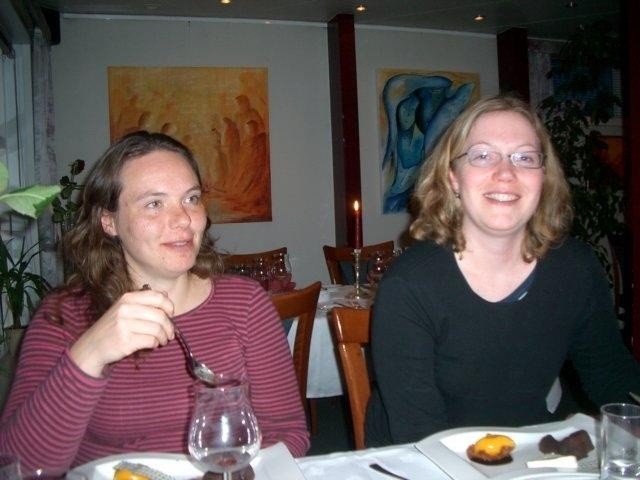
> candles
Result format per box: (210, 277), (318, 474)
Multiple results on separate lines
(353, 200), (361, 249)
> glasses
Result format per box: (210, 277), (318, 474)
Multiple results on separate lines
(451, 147), (547, 170)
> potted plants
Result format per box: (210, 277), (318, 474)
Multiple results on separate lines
(0, 230), (54, 364)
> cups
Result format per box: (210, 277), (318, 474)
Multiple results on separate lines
(0, 453), (24, 480)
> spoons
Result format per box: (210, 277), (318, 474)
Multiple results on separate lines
(141, 282), (219, 388)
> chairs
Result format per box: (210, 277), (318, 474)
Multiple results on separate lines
(322, 240), (394, 284)
(263, 280), (322, 443)
(332, 307), (372, 450)
(211, 246), (293, 296)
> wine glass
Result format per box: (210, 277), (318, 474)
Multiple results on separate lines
(366, 246), (412, 287)
(184, 372), (263, 479)
(600, 403), (639, 479)
(223, 253), (293, 294)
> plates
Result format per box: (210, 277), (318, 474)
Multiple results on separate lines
(316, 283), (343, 303)
(415, 412), (639, 479)
(64, 452), (211, 480)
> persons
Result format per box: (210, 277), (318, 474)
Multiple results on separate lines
(0, 130), (313, 479)
(361, 95), (639, 446)
